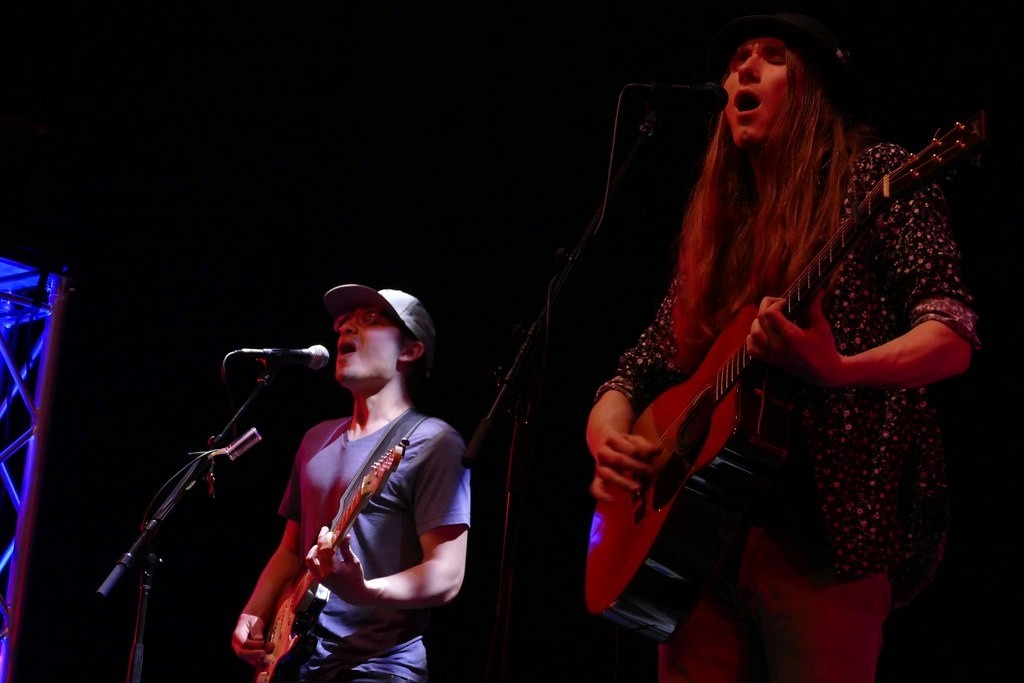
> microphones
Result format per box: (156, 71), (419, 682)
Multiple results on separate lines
(624, 81), (729, 114)
(242, 344), (329, 371)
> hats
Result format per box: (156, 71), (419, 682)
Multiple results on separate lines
(718, 11), (843, 76)
(324, 283), (434, 367)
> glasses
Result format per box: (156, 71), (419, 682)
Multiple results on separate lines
(334, 309), (404, 334)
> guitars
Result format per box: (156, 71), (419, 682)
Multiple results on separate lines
(583, 113), (988, 639)
(254, 436), (413, 683)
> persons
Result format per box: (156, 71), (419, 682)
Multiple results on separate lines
(229, 281), (470, 683)
(582, 0), (987, 683)
